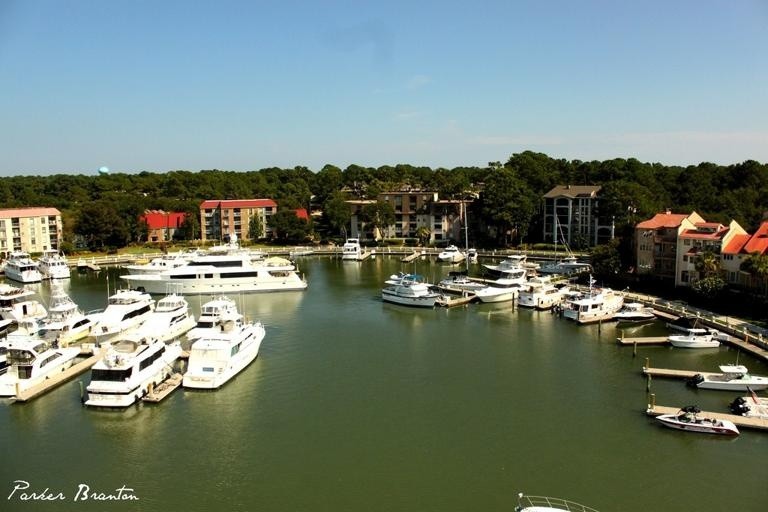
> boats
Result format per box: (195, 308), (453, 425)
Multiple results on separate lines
(3, 248), (87, 283)
(441, 268), (657, 323)
(688, 364), (768, 392)
(666, 312), (722, 348)
(382, 269), (441, 308)
(0, 284), (268, 408)
(482, 253), (539, 276)
(514, 492), (600, 512)
(438, 244), (478, 264)
(729, 385), (768, 420)
(656, 405), (741, 437)
(341, 237), (366, 259)
(118, 245), (314, 295)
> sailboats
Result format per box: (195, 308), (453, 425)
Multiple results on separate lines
(536, 213), (586, 272)
